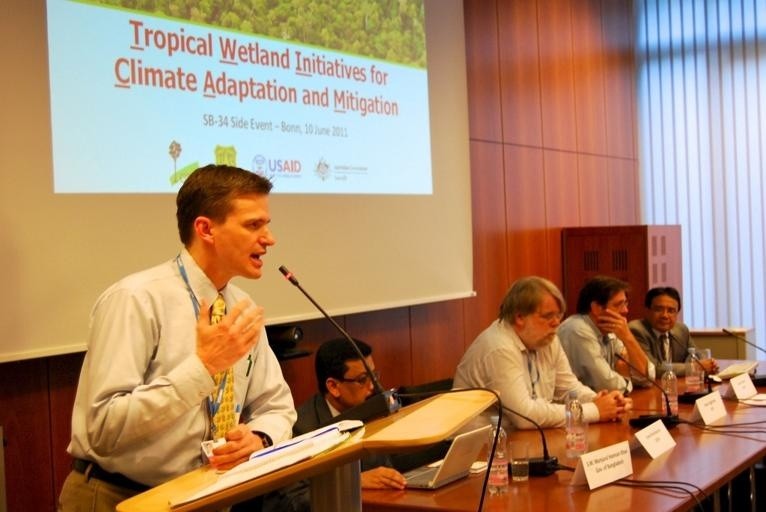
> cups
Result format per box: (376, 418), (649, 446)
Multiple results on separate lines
(508, 440), (531, 483)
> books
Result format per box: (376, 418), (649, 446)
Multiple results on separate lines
(217, 419), (366, 481)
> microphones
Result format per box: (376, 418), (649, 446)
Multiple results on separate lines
(278, 264), (402, 424)
(668, 331), (714, 404)
(613, 352), (679, 427)
(722, 328), (766, 354)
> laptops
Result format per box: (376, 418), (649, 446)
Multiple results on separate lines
(404, 424), (492, 489)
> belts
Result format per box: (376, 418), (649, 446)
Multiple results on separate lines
(73, 458), (151, 494)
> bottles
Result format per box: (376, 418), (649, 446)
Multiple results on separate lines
(565, 389), (586, 460)
(684, 348), (716, 393)
(486, 415), (509, 496)
(661, 363), (679, 417)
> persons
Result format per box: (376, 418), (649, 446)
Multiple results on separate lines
(294, 336), (409, 492)
(439, 276), (633, 456)
(554, 274), (659, 396)
(627, 286), (720, 379)
(55, 163), (299, 511)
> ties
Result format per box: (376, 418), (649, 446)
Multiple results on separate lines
(658, 335), (667, 363)
(206, 294), (238, 441)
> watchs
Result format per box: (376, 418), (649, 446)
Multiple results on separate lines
(260, 433), (273, 449)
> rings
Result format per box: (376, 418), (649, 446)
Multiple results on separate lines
(612, 324), (616, 328)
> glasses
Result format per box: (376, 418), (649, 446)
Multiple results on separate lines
(343, 370), (381, 389)
(539, 312), (564, 321)
